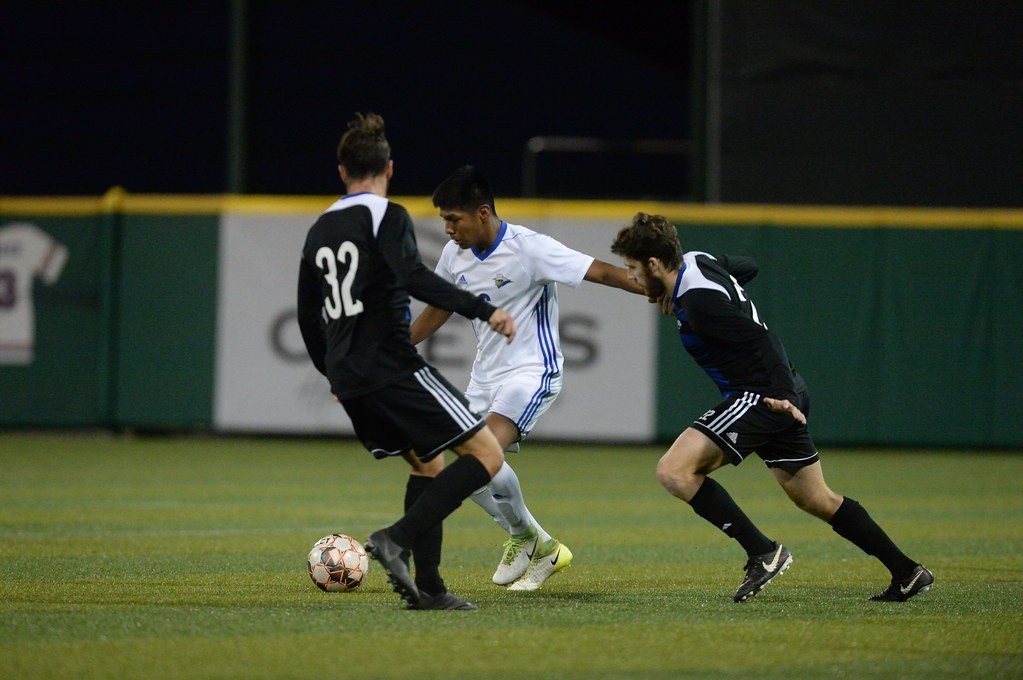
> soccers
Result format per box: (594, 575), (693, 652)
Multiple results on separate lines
(306, 534), (370, 593)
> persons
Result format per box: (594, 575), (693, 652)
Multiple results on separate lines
(609, 209), (938, 604)
(297, 108), (516, 614)
(409, 168), (649, 594)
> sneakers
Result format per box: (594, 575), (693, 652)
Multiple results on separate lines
(364, 531), (420, 606)
(869, 564), (934, 602)
(734, 541), (793, 603)
(407, 587), (479, 613)
(508, 539), (573, 591)
(492, 525), (542, 587)
